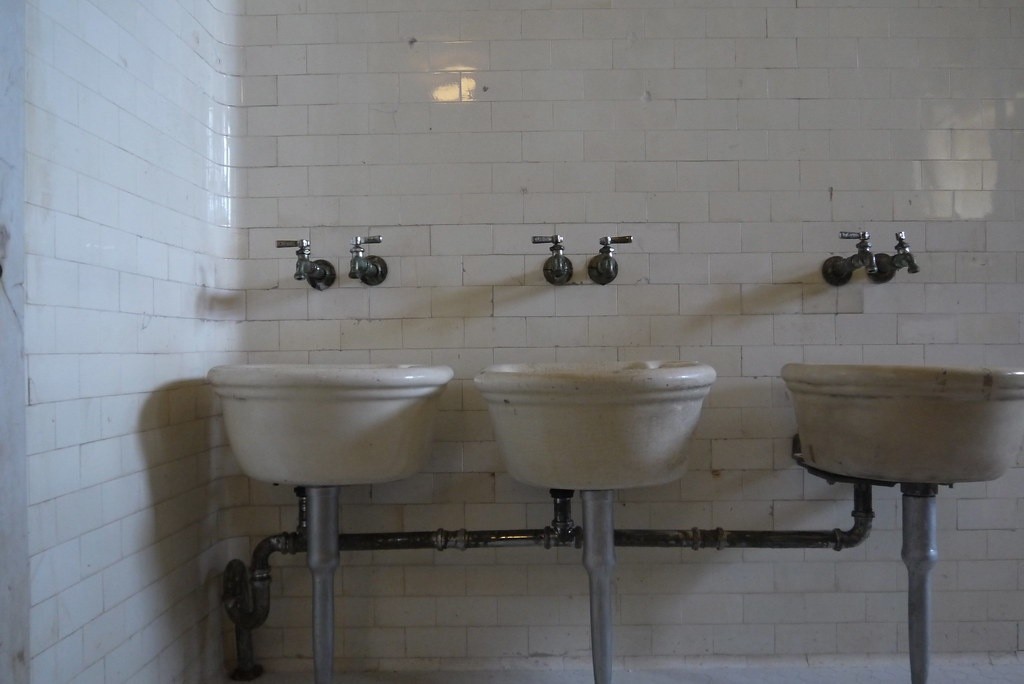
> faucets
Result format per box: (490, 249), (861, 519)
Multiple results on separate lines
(348, 235), (388, 286)
(530, 234), (574, 286)
(586, 235), (634, 286)
(822, 231), (878, 288)
(274, 238), (337, 291)
(868, 230), (920, 287)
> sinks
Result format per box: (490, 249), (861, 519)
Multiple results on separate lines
(780, 362), (1024, 484)
(206, 362), (455, 487)
(473, 360), (718, 490)
